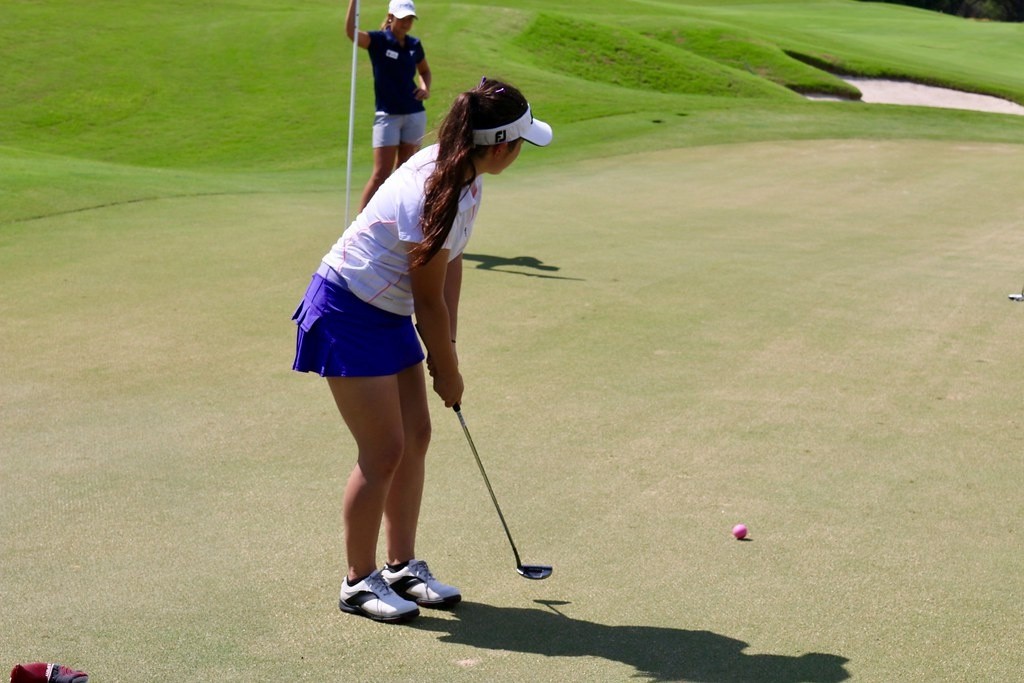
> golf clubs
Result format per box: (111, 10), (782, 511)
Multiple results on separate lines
(414, 321), (553, 580)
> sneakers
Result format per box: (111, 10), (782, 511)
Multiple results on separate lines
(337, 566), (420, 622)
(381, 559), (462, 606)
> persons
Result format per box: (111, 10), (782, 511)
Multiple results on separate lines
(295, 74), (550, 622)
(345, 0), (432, 213)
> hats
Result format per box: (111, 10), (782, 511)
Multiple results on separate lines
(388, 0), (419, 20)
(472, 99), (552, 148)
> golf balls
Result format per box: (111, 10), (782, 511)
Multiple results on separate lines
(732, 524), (748, 540)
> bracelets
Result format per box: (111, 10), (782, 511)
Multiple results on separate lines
(451, 340), (456, 343)
